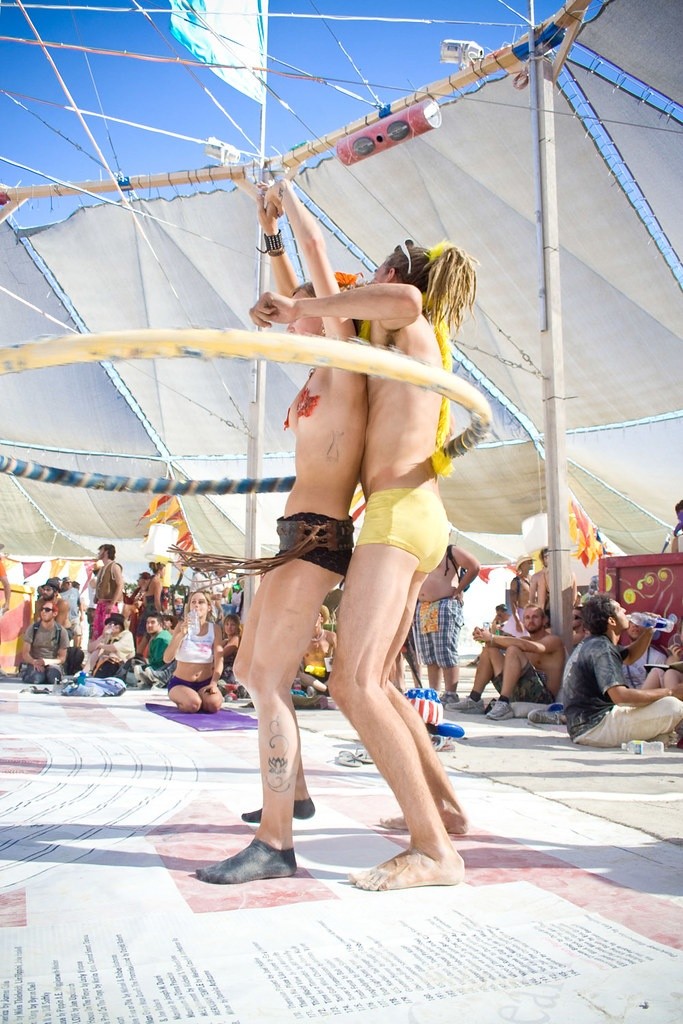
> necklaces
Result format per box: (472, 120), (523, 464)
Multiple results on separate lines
(307, 366), (317, 381)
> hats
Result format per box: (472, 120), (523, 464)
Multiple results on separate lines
(496, 604), (508, 610)
(62, 576), (72, 582)
(43, 578), (62, 591)
(105, 613), (125, 630)
(516, 554), (537, 572)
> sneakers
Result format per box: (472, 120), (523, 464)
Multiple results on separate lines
(445, 695), (486, 714)
(487, 701), (513, 720)
(439, 691), (460, 706)
(527, 708), (564, 725)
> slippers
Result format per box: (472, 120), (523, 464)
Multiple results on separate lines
(337, 750), (362, 767)
(356, 747), (373, 763)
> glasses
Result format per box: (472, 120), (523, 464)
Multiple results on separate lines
(394, 238), (415, 274)
(114, 622), (119, 625)
(575, 615), (583, 620)
(41, 608), (52, 612)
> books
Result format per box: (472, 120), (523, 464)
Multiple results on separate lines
(644, 661), (683, 673)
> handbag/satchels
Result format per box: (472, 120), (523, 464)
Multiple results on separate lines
(60, 677), (127, 698)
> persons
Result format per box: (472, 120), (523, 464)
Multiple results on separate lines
(249, 244), (476, 892)
(444, 603), (563, 720)
(293, 604), (337, 696)
(393, 544), (479, 704)
(672, 500), (683, 553)
(23, 543), (245, 688)
(528, 592), (683, 748)
(162, 593), (224, 713)
(197, 180), (368, 885)
(466, 547), (577, 668)
(0, 545), (10, 677)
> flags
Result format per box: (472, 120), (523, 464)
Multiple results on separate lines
(169, 0), (269, 103)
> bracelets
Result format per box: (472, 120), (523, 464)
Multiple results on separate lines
(491, 634), (494, 643)
(667, 687), (673, 696)
(256, 229), (286, 257)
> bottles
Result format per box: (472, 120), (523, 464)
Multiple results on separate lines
(496, 629), (499, 636)
(621, 740), (664, 754)
(187, 609), (201, 635)
(51, 677), (73, 694)
(76, 670), (93, 685)
(483, 623), (489, 631)
(626, 612), (674, 633)
(105, 621), (115, 635)
(293, 678), (301, 690)
